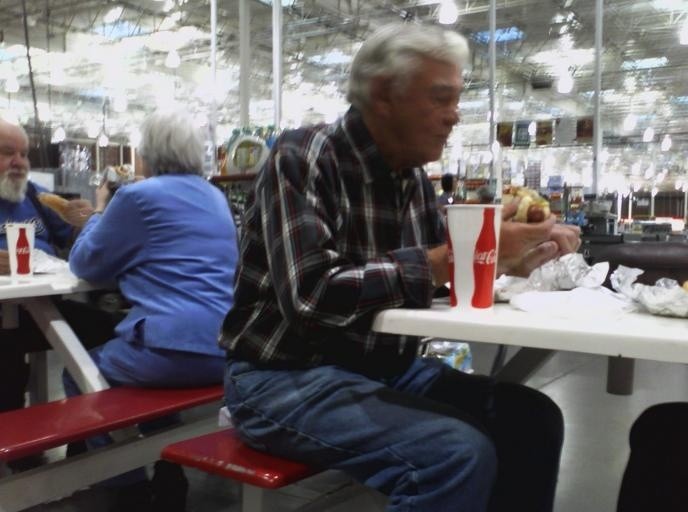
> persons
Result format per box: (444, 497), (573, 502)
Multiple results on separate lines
(438, 173), (457, 205)
(217, 21), (583, 512)
(0, 112), (92, 275)
(62, 113), (239, 512)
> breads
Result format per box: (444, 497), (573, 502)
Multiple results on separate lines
(37, 192), (71, 225)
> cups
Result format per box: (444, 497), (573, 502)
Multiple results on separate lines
(442, 201), (505, 309)
(3, 222), (36, 282)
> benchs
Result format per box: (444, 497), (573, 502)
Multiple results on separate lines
(0, 385), (330, 511)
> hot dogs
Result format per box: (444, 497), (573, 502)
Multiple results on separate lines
(500, 187), (551, 223)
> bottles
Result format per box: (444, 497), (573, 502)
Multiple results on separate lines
(223, 181), (248, 214)
(228, 123), (277, 151)
